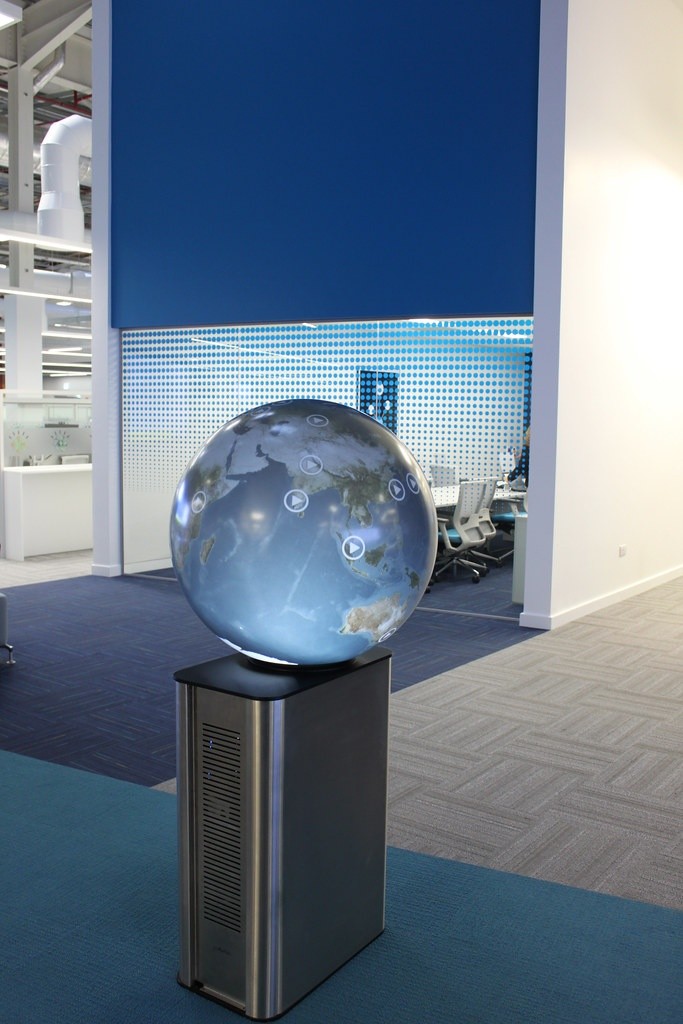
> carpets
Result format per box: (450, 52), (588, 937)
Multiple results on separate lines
(0, 549), (683, 1024)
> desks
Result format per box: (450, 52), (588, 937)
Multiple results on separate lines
(431, 485), (524, 508)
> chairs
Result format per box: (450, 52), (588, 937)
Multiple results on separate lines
(424, 454), (528, 593)
